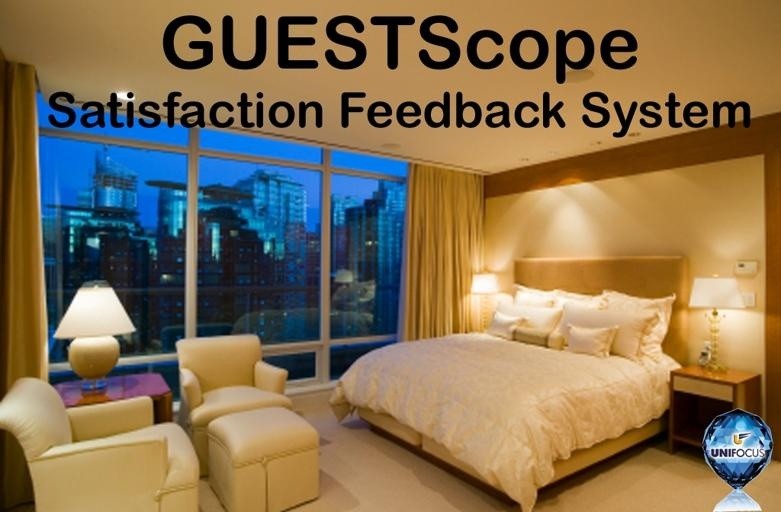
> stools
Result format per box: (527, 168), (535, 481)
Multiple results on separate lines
(207, 407), (324, 512)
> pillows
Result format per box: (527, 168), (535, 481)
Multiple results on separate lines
(486, 281), (681, 366)
(332, 281), (376, 310)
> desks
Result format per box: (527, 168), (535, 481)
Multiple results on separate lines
(57, 372), (175, 424)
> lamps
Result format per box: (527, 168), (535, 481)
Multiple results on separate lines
(332, 267), (356, 286)
(52, 280), (138, 391)
(689, 275), (751, 378)
(470, 269), (500, 332)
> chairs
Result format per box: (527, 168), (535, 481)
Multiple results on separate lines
(0, 374), (201, 511)
(174, 332), (296, 476)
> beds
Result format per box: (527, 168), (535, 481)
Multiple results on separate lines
(326, 254), (692, 510)
(228, 306), (374, 343)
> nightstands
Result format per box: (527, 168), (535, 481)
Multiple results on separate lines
(667, 366), (761, 469)
